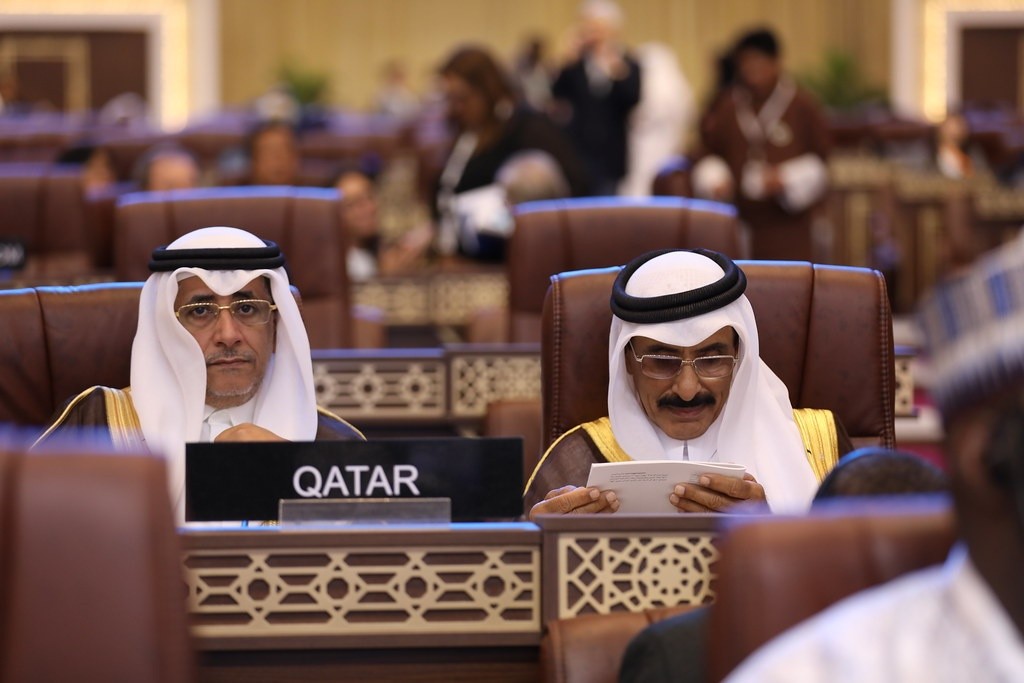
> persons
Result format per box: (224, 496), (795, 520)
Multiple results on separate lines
(33, 225), (367, 532)
(609, 329), (1023, 683)
(522, 248), (822, 524)
(54, 1), (998, 259)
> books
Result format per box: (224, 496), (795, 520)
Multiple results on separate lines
(586, 459), (746, 514)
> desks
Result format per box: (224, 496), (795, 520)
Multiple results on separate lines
(176, 511), (745, 683)
(314, 343), (541, 444)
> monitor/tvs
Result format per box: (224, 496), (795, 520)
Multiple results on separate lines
(185, 438), (524, 529)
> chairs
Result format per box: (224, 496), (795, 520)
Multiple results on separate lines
(0, 104), (424, 683)
(416, 113), (1024, 683)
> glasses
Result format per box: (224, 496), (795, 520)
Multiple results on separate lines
(174, 299), (277, 332)
(629, 341), (739, 380)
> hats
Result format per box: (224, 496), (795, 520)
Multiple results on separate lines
(919, 233), (1024, 395)
